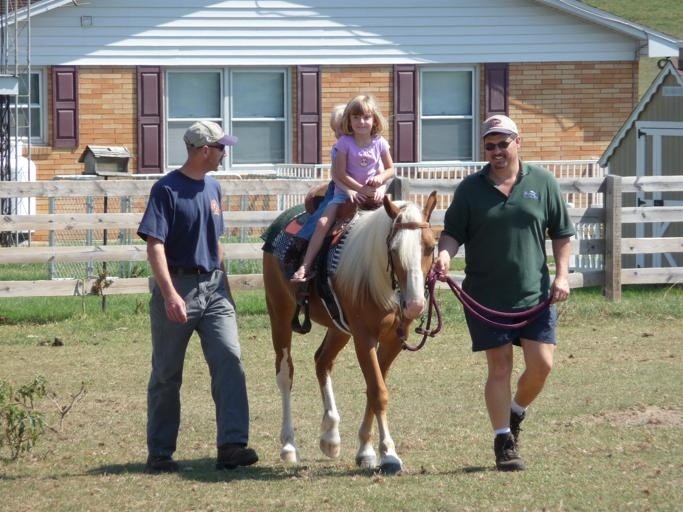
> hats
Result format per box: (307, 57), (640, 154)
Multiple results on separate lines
(480, 114), (519, 139)
(183, 121), (240, 151)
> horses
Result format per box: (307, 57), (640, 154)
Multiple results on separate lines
(261, 190), (439, 475)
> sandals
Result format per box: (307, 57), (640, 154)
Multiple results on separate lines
(289, 263), (316, 285)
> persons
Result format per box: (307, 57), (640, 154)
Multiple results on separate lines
(135, 119), (259, 471)
(432, 113), (576, 471)
(282, 104), (387, 264)
(289, 94), (396, 284)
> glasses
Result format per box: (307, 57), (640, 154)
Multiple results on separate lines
(483, 140), (515, 151)
(207, 143), (225, 150)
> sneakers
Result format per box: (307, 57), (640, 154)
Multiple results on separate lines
(492, 431), (526, 474)
(145, 455), (195, 476)
(212, 446), (260, 471)
(509, 407), (527, 444)
